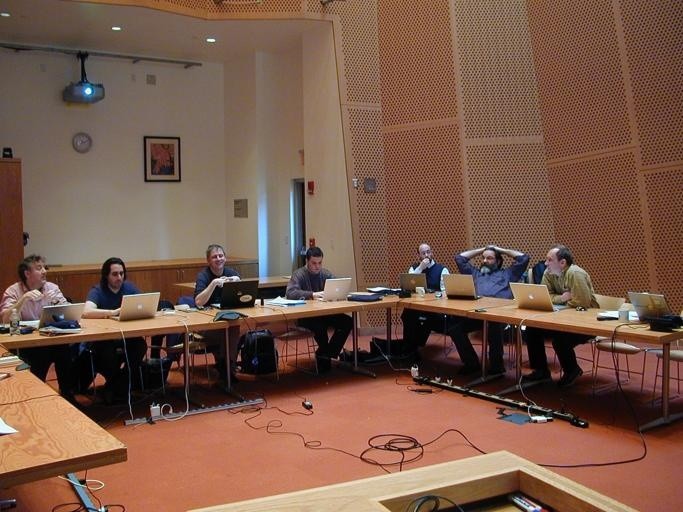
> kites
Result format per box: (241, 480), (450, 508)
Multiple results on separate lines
(510, 493), (550, 512)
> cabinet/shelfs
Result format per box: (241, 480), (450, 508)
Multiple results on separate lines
(8, 309), (20, 337)
(46, 257), (258, 306)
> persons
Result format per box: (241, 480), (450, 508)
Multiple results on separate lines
(193, 244), (241, 384)
(151, 144), (173, 175)
(286, 247), (354, 377)
(521, 244), (600, 386)
(448, 244), (531, 379)
(85, 258), (147, 395)
(0, 254), (81, 398)
(402, 243), (450, 351)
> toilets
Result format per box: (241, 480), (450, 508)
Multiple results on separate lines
(214, 310), (250, 323)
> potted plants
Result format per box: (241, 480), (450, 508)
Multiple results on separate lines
(145, 136), (181, 181)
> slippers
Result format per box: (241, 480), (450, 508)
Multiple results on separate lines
(238, 328), (278, 374)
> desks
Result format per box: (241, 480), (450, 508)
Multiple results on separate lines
(0, 274), (683, 511)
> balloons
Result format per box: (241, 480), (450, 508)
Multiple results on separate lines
(73, 133), (92, 153)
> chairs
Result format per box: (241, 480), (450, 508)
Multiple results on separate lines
(458, 366), (583, 387)
(316, 349), (339, 361)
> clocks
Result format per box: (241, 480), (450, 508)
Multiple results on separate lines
(73, 133), (92, 153)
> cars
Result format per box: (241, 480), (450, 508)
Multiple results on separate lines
(618, 309), (630, 323)
(433, 291), (442, 299)
(415, 286), (425, 298)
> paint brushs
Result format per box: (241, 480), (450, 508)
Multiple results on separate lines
(125, 398), (263, 424)
(415, 373), (573, 421)
(65, 473), (107, 512)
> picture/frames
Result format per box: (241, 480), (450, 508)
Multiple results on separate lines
(145, 136), (181, 181)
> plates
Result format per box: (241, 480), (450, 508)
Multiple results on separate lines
(46, 257), (258, 306)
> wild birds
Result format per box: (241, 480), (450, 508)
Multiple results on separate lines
(108, 292), (161, 321)
(443, 273), (479, 299)
(627, 292), (670, 320)
(509, 282), (565, 311)
(209, 280), (258, 309)
(399, 273), (431, 293)
(36, 302), (86, 331)
(316, 278), (352, 301)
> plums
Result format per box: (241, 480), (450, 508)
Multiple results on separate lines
(64, 82), (106, 105)
